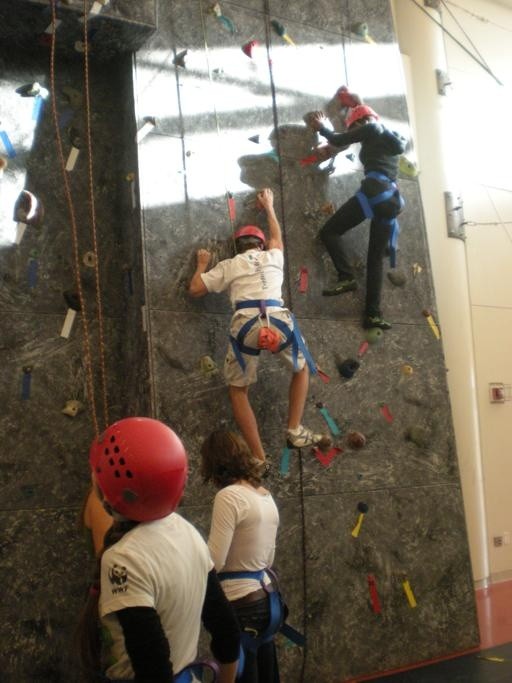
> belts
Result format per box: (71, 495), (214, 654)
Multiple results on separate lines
(239, 582), (274, 604)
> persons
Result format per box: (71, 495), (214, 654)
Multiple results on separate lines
(188, 186), (333, 482)
(76, 414), (244, 681)
(191, 425), (287, 682)
(306, 90), (404, 330)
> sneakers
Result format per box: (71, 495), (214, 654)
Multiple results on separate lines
(251, 457), (272, 481)
(364, 316), (392, 330)
(323, 278), (358, 297)
(286, 425), (324, 448)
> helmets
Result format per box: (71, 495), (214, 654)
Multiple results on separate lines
(90, 417), (188, 521)
(346, 104), (379, 128)
(234, 226), (265, 242)
